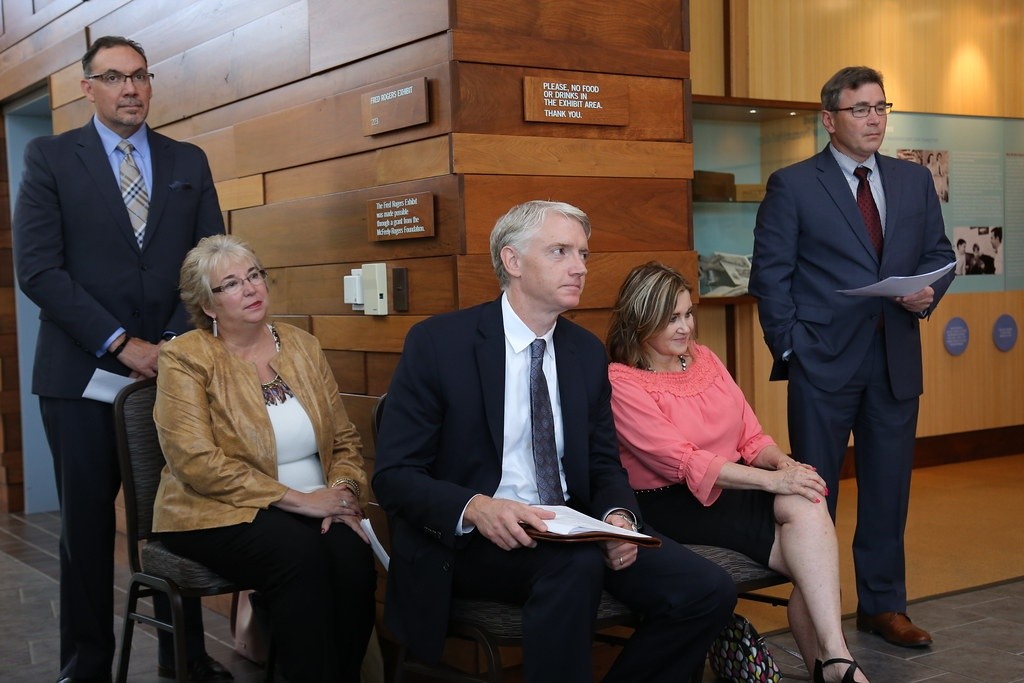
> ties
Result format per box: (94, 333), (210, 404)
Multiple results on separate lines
(116, 140), (149, 251)
(853, 166), (884, 261)
(531, 339), (565, 506)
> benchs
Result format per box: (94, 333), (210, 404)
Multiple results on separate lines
(371, 389), (794, 683)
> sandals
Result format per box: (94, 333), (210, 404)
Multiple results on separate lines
(813, 658), (870, 683)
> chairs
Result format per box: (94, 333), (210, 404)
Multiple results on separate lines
(114, 378), (380, 683)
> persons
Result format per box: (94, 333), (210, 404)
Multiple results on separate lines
(969, 243), (985, 274)
(936, 153), (943, 176)
(370, 201), (738, 683)
(151, 235), (377, 683)
(14, 36), (233, 683)
(927, 154), (934, 177)
(748, 66), (957, 645)
(991, 227), (1003, 274)
(954, 239), (967, 275)
(605, 261), (870, 683)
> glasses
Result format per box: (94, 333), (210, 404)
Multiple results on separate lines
(211, 269), (267, 294)
(87, 71), (154, 84)
(827, 103), (893, 118)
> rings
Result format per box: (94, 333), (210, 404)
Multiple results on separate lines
(343, 500), (348, 508)
(619, 557), (623, 565)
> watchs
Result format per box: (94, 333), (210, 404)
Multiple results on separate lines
(161, 333), (175, 341)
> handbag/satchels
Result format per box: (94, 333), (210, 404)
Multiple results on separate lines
(709, 612), (782, 683)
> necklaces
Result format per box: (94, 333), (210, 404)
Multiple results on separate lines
(647, 354), (686, 372)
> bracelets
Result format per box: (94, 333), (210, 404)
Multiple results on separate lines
(332, 478), (360, 496)
(615, 513), (638, 533)
(112, 335), (130, 356)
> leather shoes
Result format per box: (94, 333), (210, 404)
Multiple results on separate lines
(857, 601), (933, 646)
(56, 677), (73, 683)
(158, 656), (233, 683)
